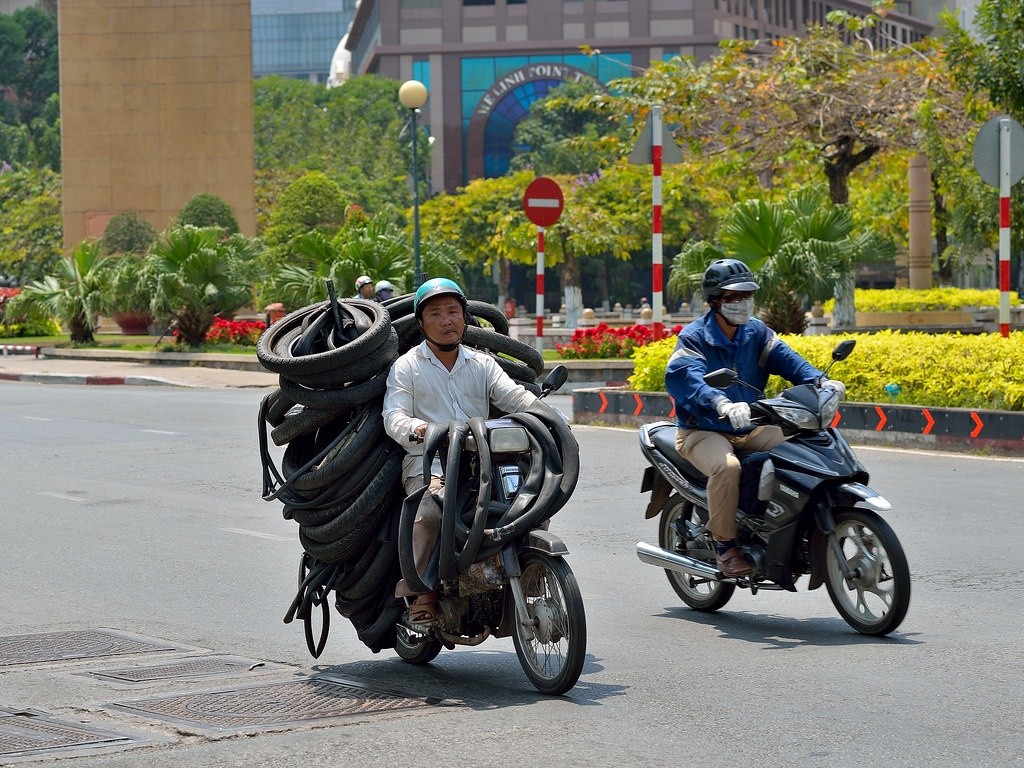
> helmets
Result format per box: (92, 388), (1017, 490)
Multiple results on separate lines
(354, 275), (373, 292)
(374, 280), (394, 296)
(703, 259), (760, 302)
(414, 278), (467, 317)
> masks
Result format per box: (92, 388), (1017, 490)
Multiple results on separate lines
(719, 301), (754, 324)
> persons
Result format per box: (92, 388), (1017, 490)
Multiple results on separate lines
(634, 297), (650, 314)
(664, 259), (846, 577)
(673, 294), (684, 312)
(381, 278), (569, 623)
(352, 276), (396, 303)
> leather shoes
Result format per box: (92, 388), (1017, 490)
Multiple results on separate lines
(710, 539), (751, 577)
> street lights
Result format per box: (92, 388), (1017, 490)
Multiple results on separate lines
(398, 79), (428, 292)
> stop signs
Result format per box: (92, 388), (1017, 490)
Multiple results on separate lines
(524, 177), (564, 227)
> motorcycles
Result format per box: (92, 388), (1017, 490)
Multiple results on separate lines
(334, 365), (587, 694)
(636, 339), (911, 636)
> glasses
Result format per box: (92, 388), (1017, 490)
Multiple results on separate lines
(720, 291), (751, 305)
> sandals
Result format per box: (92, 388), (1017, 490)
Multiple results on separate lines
(408, 598), (436, 623)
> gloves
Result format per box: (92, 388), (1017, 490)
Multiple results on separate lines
(822, 377), (846, 400)
(716, 400), (752, 430)
